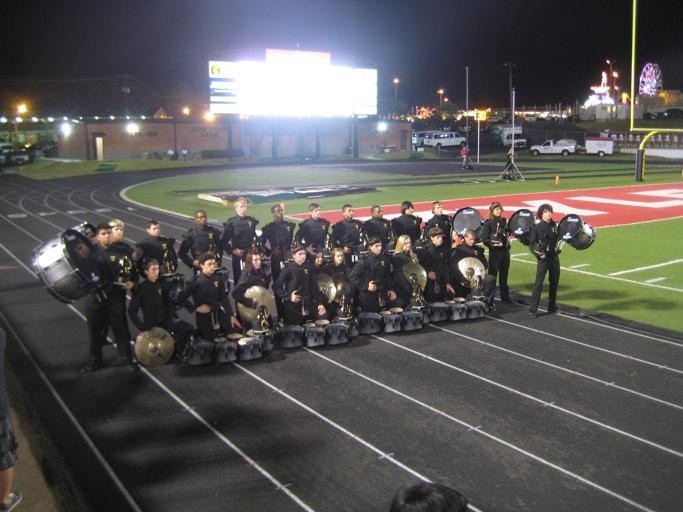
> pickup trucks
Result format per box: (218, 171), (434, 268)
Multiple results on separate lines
(530, 138), (579, 156)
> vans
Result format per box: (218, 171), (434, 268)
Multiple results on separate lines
(424, 132), (467, 148)
(411, 130), (443, 147)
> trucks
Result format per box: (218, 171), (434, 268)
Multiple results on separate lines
(493, 123), (527, 149)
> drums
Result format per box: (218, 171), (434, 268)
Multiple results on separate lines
(190, 328), (274, 364)
(32, 222), (108, 304)
(557, 213), (596, 249)
(277, 313), (359, 350)
(430, 295), (487, 323)
(162, 272), (184, 299)
(215, 266), (231, 292)
(359, 304), (430, 333)
(452, 207), (483, 243)
(509, 209), (540, 246)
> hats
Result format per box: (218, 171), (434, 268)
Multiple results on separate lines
(290, 244), (304, 254)
(427, 227), (446, 237)
(199, 252), (215, 263)
(368, 236), (380, 246)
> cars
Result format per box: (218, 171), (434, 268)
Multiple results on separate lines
(0, 136), (56, 168)
(653, 108), (682, 119)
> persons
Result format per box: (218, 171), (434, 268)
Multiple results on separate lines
(0, 325), (23, 512)
(84, 196), (514, 371)
(459, 141), (470, 168)
(530, 204), (562, 318)
(388, 481), (469, 512)
(505, 145), (517, 178)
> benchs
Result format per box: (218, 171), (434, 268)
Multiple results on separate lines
(197, 147), (245, 162)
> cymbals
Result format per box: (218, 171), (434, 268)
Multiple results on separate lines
(237, 285), (273, 323)
(134, 327), (174, 366)
(317, 273), (348, 304)
(458, 256), (486, 288)
(403, 262), (427, 291)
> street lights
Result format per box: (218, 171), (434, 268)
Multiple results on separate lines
(392, 78), (401, 117)
(503, 61), (516, 119)
(605, 60), (622, 114)
(18, 102), (28, 142)
(438, 88), (445, 116)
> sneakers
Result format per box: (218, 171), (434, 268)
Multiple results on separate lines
(0, 489), (22, 512)
